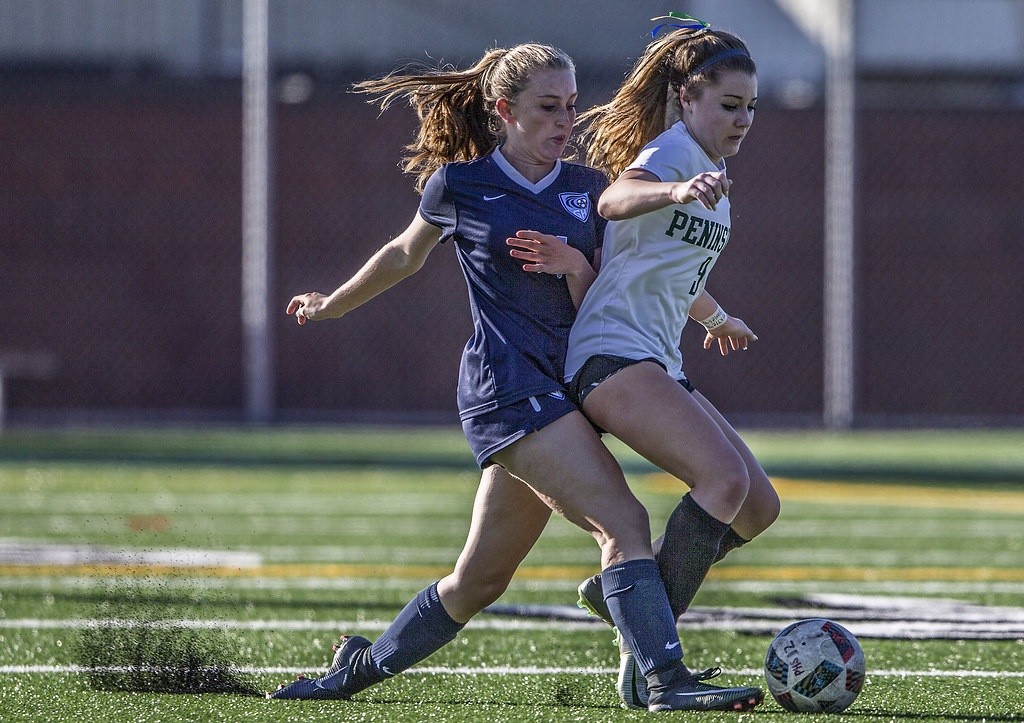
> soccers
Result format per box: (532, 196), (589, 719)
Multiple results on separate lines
(765, 618), (864, 713)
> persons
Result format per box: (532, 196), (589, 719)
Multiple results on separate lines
(563, 14), (782, 704)
(266, 44), (765, 712)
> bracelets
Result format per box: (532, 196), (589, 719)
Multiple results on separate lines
(700, 304), (728, 330)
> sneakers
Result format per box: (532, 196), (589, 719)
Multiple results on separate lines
(267, 634), (372, 699)
(613, 626), (650, 711)
(646, 660), (765, 713)
(576, 574), (616, 629)
